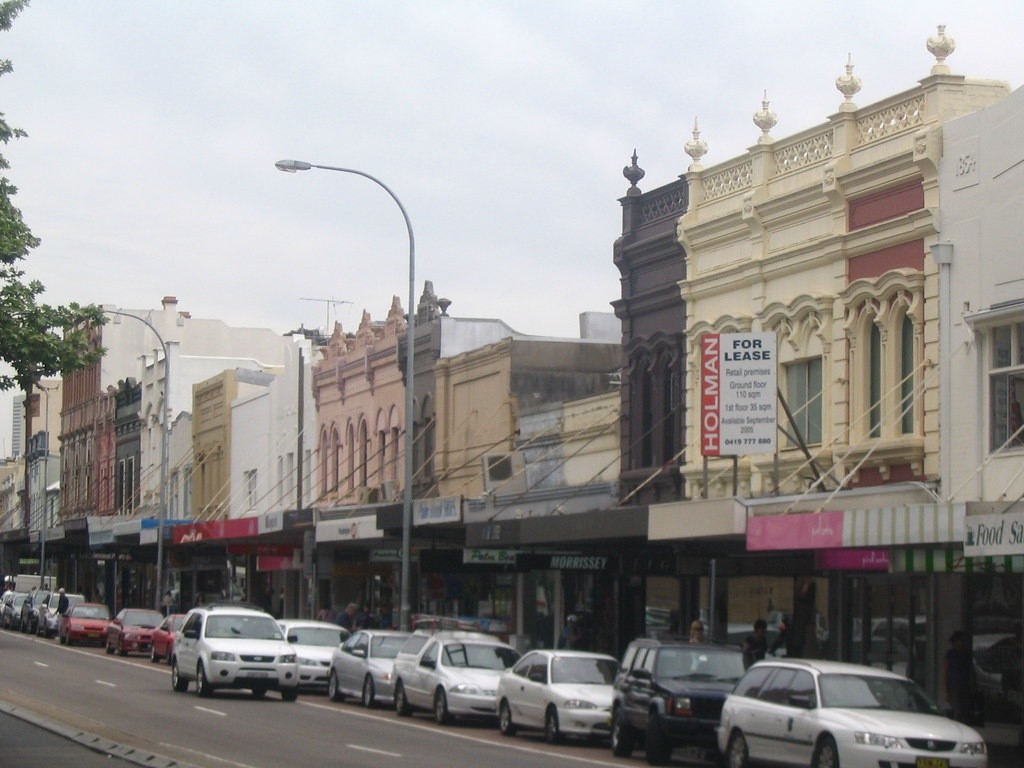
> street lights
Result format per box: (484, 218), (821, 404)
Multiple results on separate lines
(275, 160), (415, 632)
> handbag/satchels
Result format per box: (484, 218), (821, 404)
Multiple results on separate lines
(50, 614), (59, 629)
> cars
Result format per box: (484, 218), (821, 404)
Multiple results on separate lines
(105, 608), (164, 656)
(724, 624), (787, 656)
(495, 650), (620, 745)
(60, 603), (113, 647)
(20, 590), (49, 633)
(716, 657), (987, 768)
(327, 630), (412, 707)
(852, 618), (927, 643)
(36, 592), (86, 638)
(150, 613), (187, 665)
(391, 629), (524, 726)
(0, 590), (29, 631)
(275, 619), (353, 686)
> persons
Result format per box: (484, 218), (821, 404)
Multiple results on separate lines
(316, 599), (380, 632)
(942, 631), (978, 723)
(52, 588), (69, 640)
(563, 614), (582, 650)
(689, 620), (708, 644)
(741, 619), (767, 672)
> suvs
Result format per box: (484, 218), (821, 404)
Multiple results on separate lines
(609, 634), (755, 765)
(172, 602), (300, 703)
